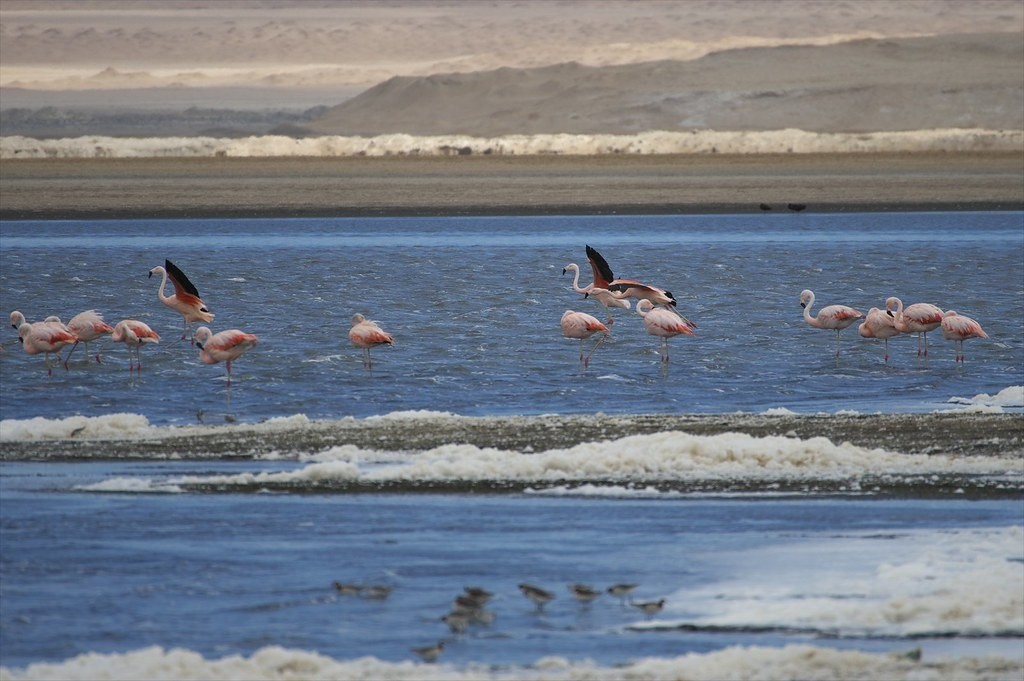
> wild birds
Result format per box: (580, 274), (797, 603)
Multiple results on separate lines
(10, 244), (990, 395)
(334, 581), (921, 663)
